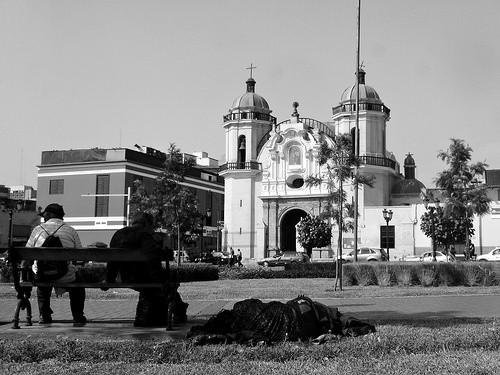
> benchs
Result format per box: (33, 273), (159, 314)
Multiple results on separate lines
(7, 244), (181, 331)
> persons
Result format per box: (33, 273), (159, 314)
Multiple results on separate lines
(464, 239), (474, 261)
(105, 213), (189, 326)
(227, 247), (244, 267)
(19, 203), (88, 324)
(297, 252), (309, 262)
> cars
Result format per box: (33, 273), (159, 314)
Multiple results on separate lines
(475, 246), (500, 262)
(403, 250), (453, 263)
(257, 251), (310, 268)
(333, 247), (388, 264)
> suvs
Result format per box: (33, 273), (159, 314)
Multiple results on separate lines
(172, 250), (193, 263)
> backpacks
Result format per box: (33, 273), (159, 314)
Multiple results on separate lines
(37, 224), (70, 282)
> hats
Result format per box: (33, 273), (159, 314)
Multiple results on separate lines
(37, 204), (64, 217)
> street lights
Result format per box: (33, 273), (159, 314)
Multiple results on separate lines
(197, 208), (212, 257)
(0, 198), (25, 267)
(382, 208), (394, 261)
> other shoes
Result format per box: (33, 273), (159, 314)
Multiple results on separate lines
(173, 303), (189, 317)
(39, 315), (52, 323)
(134, 318), (168, 327)
(73, 316), (86, 327)
